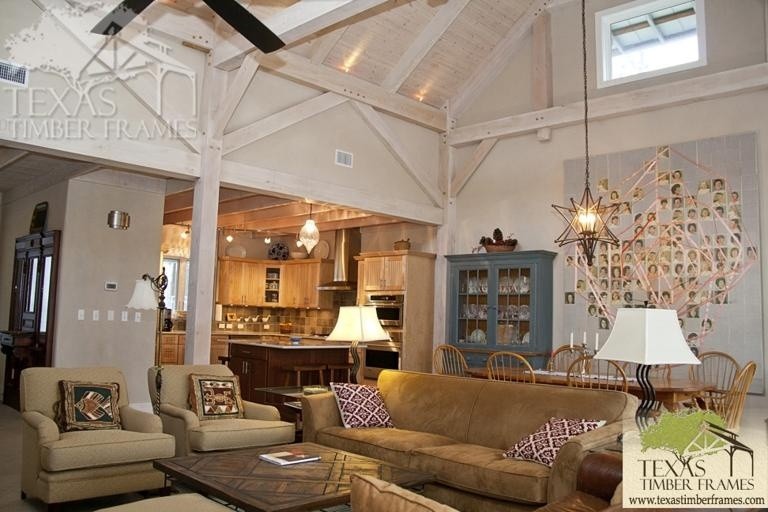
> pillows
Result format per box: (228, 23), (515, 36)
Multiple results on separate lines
(346, 472), (462, 512)
(609, 476), (625, 505)
(501, 415), (608, 468)
(57, 377), (125, 434)
(327, 378), (397, 431)
(187, 372), (246, 422)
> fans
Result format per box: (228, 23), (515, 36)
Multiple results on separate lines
(87, 1), (289, 57)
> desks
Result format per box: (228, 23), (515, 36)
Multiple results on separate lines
(586, 439), (625, 459)
(250, 381), (333, 442)
(464, 364), (719, 415)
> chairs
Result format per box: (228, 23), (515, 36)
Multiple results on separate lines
(545, 342), (592, 374)
(146, 360), (298, 457)
(688, 358), (758, 432)
(483, 348), (538, 384)
(619, 365), (672, 429)
(16, 364), (177, 511)
(535, 450), (687, 511)
(565, 354), (628, 393)
(430, 344), (471, 379)
(683, 349), (742, 414)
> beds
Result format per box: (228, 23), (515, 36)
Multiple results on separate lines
(153, 440), (439, 511)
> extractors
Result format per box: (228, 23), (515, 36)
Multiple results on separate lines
(312, 228), (358, 293)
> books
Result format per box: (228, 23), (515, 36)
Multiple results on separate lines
(260, 452), (321, 466)
(302, 385), (327, 394)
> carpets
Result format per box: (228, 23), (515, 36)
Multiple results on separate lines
(171, 478), (424, 511)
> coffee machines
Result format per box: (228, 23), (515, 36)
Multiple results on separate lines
(162, 308), (173, 331)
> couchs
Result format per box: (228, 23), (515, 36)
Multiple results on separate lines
(300, 365), (641, 511)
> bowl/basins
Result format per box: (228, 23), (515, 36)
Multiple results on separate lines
(291, 251), (308, 259)
(484, 245), (516, 253)
(289, 337), (303, 345)
(393, 241), (411, 250)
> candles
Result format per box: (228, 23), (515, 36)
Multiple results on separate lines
(569, 331), (574, 349)
(582, 331), (586, 344)
(595, 332), (599, 349)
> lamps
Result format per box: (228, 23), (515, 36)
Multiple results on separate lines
(548, 0), (623, 268)
(295, 203), (321, 251)
(125, 267), (170, 412)
(323, 304), (392, 384)
(589, 306), (703, 436)
(180, 224), (275, 246)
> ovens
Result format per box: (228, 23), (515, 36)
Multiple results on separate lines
(361, 293), (404, 381)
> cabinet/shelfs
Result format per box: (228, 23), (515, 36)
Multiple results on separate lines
(442, 248), (559, 378)
(217, 254), (260, 308)
(158, 333), (178, 365)
(0, 228), (64, 411)
(178, 334), (187, 365)
(209, 334), (229, 366)
(224, 336), (370, 418)
(285, 258), (336, 312)
(359, 248), (437, 291)
(259, 256), (285, 309)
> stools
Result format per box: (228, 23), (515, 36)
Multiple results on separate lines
(323, 362), (355, 385)
(216, 353), (228, 366)
(95, 490), (239, 512)
(280, 363), (327, 429)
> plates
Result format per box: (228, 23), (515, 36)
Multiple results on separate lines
(459, 271), (529, 346)
(312, 239), (330, 260)
(267, 241), (289, 261)
(225, 243), (248, 259)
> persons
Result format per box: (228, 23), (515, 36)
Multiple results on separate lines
(562, 145), (742, 359)
